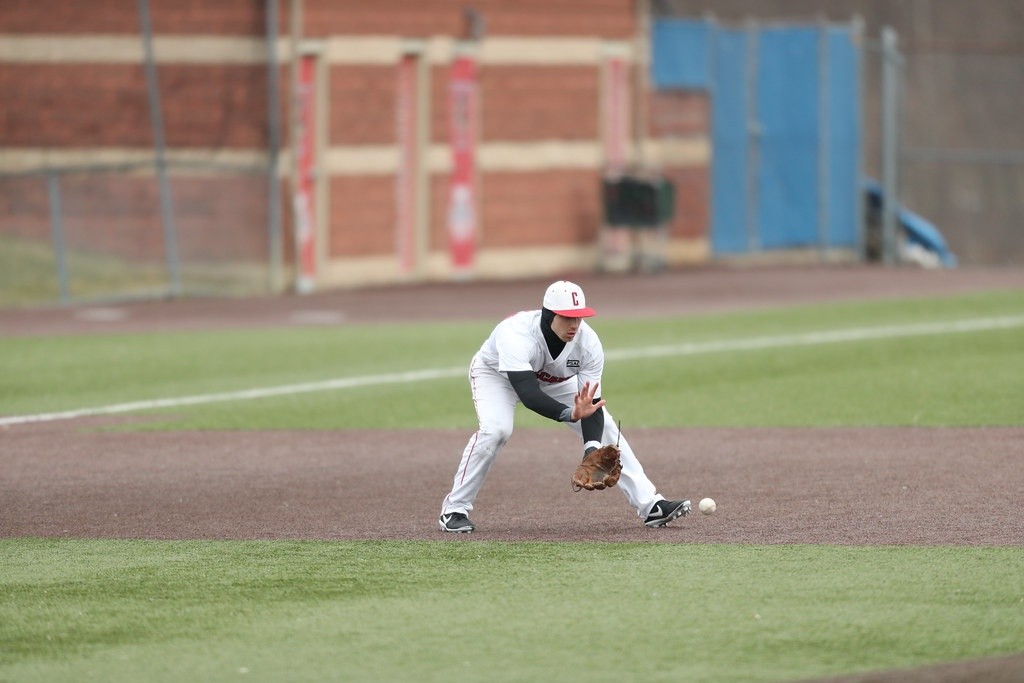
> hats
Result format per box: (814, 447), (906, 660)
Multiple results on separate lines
(543, 280), (596, 317)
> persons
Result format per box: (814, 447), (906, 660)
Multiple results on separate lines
(436, 277), (689, 536)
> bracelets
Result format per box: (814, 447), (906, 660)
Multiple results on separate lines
(583, 440), (601, 451)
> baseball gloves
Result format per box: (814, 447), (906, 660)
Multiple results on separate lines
(572, 444), (623, 492)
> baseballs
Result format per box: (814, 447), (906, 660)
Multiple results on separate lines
(699, 498), (717, 515)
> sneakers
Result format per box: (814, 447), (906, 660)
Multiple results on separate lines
(644, 499), (691, 528)
(439, 512), (475, 534)
